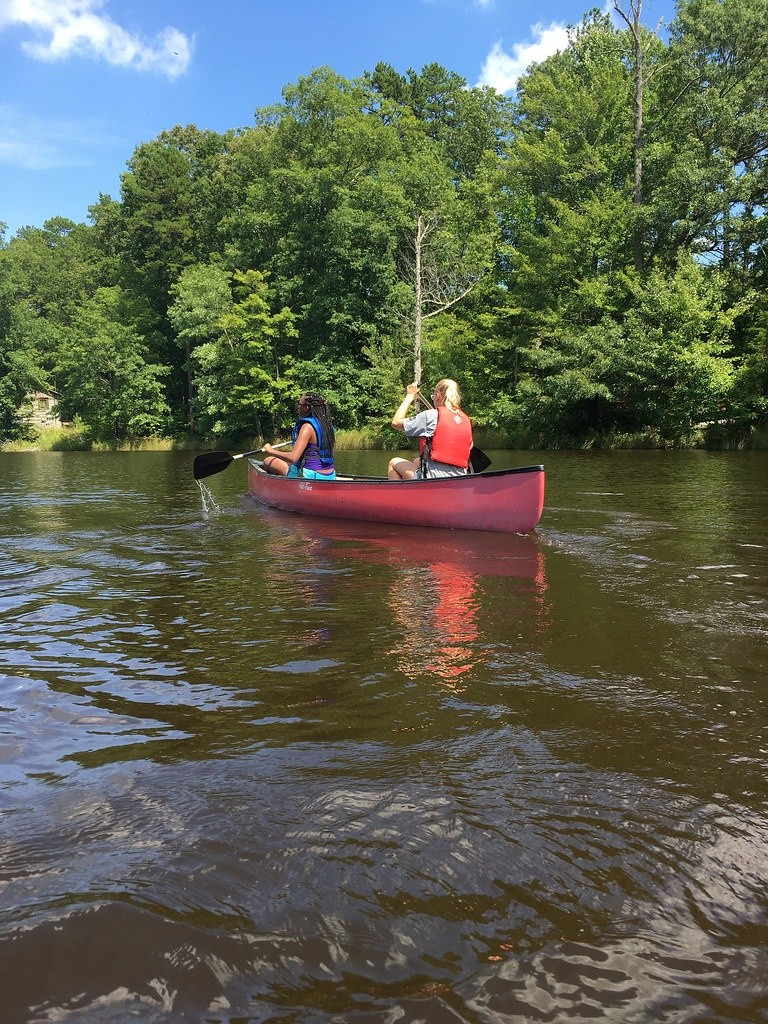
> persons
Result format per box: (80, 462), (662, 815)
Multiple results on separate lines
(387, 378), (473, 480)
(263, 391), (336, 481)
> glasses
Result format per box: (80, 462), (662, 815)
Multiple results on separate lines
(430, 394), (436, 399)
(295, 401), (303, 406)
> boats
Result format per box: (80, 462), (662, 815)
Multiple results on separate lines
(246, 458), (545, 533)
